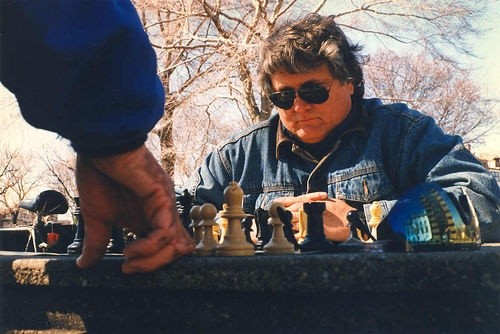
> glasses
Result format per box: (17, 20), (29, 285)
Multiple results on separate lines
(267, 76), (336, 110)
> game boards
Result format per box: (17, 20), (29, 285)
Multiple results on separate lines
(9, 180), (368, 251)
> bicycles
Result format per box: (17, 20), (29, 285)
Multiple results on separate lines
(18, 190), (70, 253)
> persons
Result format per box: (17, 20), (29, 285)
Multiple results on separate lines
(1, 0), (196, 273)
(196, 14), (500, 244)
